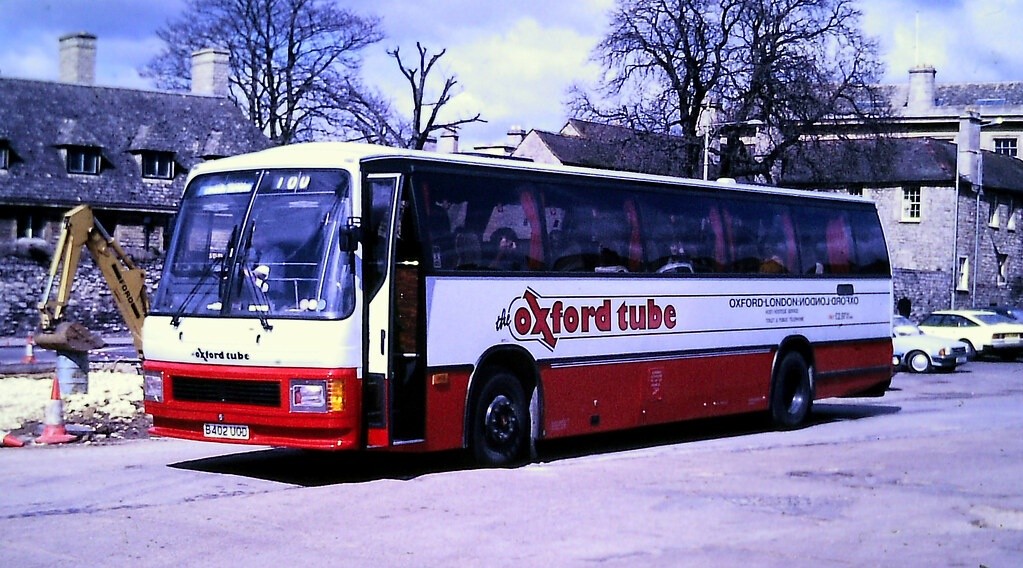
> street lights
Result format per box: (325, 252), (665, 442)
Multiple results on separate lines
(703, 116), (762, 181)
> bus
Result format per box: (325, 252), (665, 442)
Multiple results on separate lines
(141, 141), (893, 470)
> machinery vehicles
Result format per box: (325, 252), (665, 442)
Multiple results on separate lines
(29, 203), (149, 364)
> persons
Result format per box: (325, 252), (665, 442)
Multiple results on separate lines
(422, 184), (455, 271)
(245, 229), (288, 299)
(545, 229), (583, 273)
(802, 233), (824, 275)
(758, 239), (787, 277)
(897, 294), (911, 318)
(662, 237), (694, 275)
(481, 228), (527, 274)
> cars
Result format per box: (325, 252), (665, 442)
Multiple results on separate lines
(917, 307), (1023, 362)
(893, 313), (970, 374)
(940, 304), (1023, 327)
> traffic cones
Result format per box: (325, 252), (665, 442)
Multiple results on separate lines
(33, 370), (78, 444)
(0, 431), (25, 449)
(21, 330), (40, 364)
